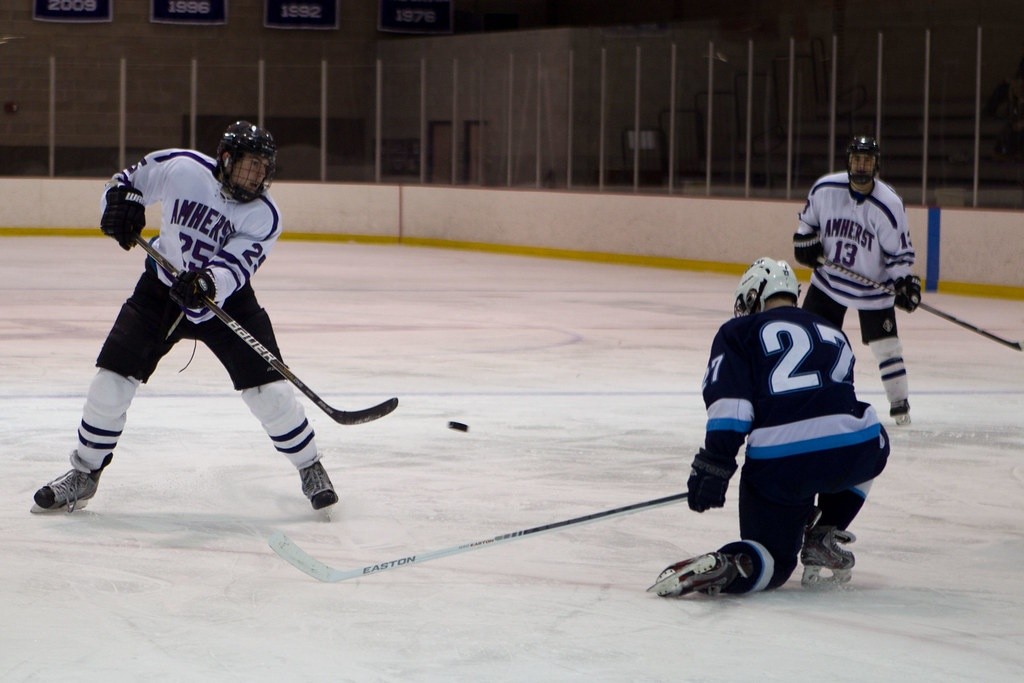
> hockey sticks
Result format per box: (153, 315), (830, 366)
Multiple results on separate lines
(820, 257), (1024, 351)
(268, 492), (693, 585)
(137, 235), (400, 427)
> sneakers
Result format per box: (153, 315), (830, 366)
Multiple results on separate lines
(30, 448), (115, 513)
(801, 527), (854, 586)
(299, 462), (338, 522)
(646, 552), (741, 598)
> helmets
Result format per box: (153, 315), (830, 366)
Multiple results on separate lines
(217, 121), (277, 203)
(740, 257), (798, 313)
(846, 134), (880, 187)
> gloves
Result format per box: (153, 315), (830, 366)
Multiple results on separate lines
(169, 269), (215, 306)
(686, 446), (738, 512)
(793, 232), (826, 269)
(100, 185), (146, 250)
(894, 275), (921, 312)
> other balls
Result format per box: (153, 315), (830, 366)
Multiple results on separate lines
(447, 420), (470, 433)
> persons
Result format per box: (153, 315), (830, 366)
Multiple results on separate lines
(653, 255), (892, 601)
(30, 121), (339, 511)
(790, 133), (921, 421)
(990, 49), (1024, 160)
